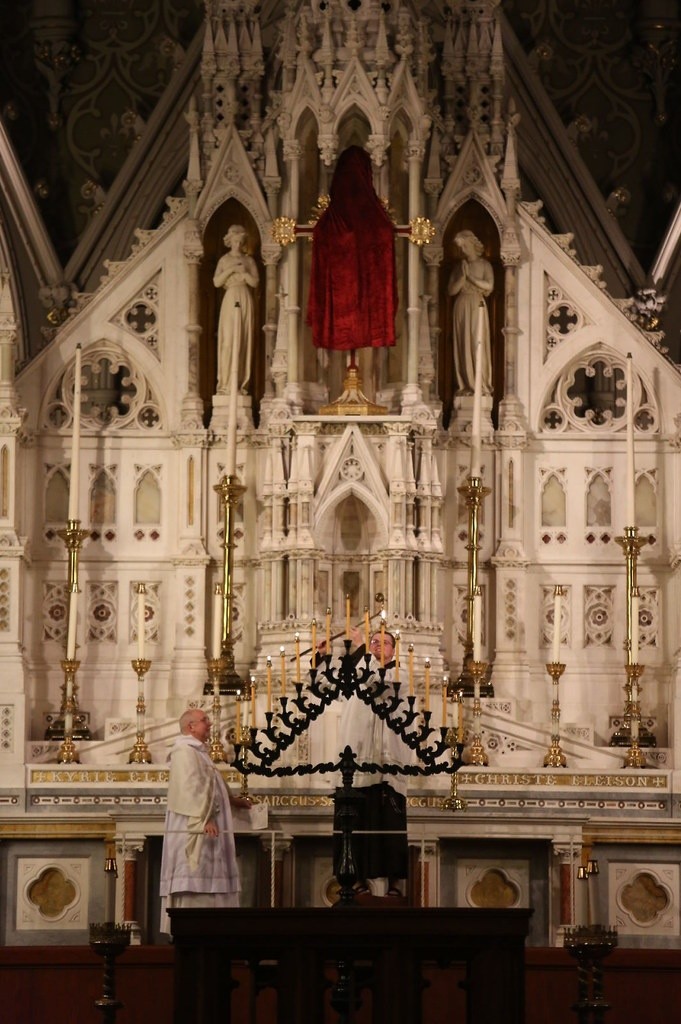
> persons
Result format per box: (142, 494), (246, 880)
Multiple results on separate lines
(213, 225), (258, 396)
(161, 710), (253, 937)
(448, 231), (494, 398)
(310, 631), (415, 898)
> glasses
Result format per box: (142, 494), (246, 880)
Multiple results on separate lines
(371, 639), (392, 646)
(189, 718), (210, 723)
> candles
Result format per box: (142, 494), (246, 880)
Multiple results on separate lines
(235, 593), (463, 744)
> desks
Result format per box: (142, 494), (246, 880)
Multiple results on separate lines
(166, 906), (535, 1024)
